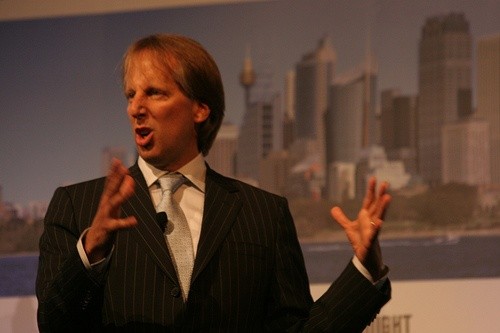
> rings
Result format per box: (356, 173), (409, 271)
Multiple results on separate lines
(370, 221), (375, 227)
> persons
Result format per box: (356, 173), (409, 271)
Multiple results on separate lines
(35, 33), (392, 333)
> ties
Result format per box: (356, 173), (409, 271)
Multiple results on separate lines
(154, 172), (196, 302)
